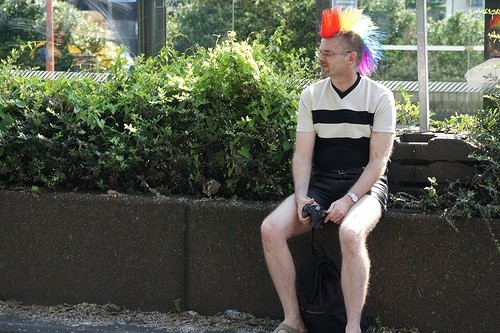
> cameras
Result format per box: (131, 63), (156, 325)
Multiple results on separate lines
(302, 205), (328, 229)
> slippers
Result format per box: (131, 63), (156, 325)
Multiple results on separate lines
(271, 322), (308, 333)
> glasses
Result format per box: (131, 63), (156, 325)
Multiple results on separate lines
(315, 50), (349, 58)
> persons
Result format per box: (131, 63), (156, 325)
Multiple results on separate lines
(260, 7), (398, 333)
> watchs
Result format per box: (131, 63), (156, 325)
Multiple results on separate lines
(347, 190), (359, 204)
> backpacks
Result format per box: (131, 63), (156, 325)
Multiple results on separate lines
(297, 238), (371, 333)
